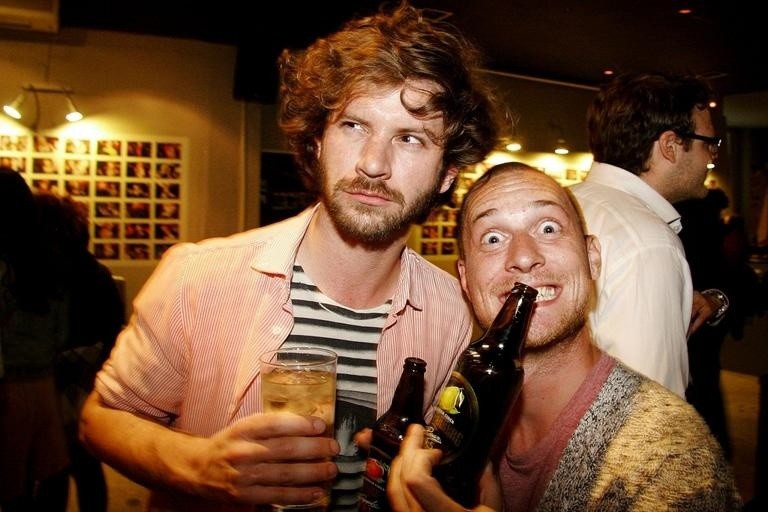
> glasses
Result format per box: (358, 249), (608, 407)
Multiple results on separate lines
(684, 134), (722, 153)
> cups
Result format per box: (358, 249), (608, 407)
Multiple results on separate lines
(258, 346), (339, 510)
(700, 288), (730, 326)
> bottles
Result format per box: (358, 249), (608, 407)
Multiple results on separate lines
(356, 356), (428, 510)
(422, 281), (540, 509)
